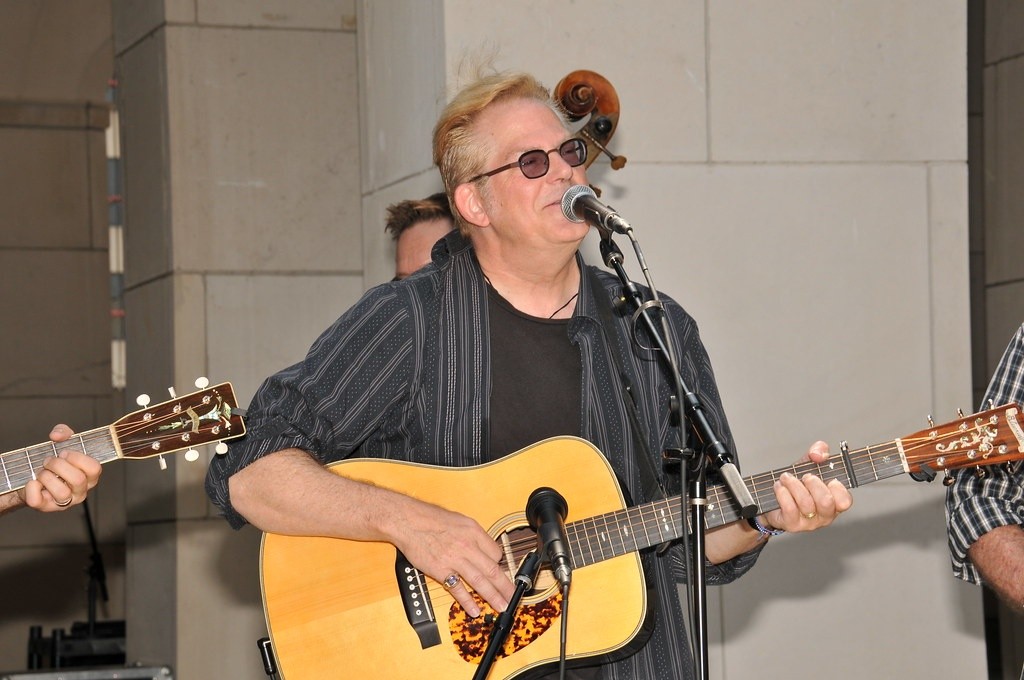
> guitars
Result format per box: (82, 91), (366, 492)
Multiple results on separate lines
(0, 375), (248, 495)
(259, 397), (1024, 679)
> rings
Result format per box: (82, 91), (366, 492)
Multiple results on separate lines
(56, 497), (74, 509)
(802, 510), (819, 521)
(438, 572), (466, 591)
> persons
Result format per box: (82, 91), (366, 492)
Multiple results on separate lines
(1, 419), (108, 534)
(375, 190), (464, 287)
(198, 69), (861, 679)
(943, 312), (1024, 618)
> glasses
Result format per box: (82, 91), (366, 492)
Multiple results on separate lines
(467, 137), (588, 188)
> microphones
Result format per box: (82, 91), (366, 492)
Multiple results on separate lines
(560, 185), (633, 234)
(526, 487), (572, 585)
